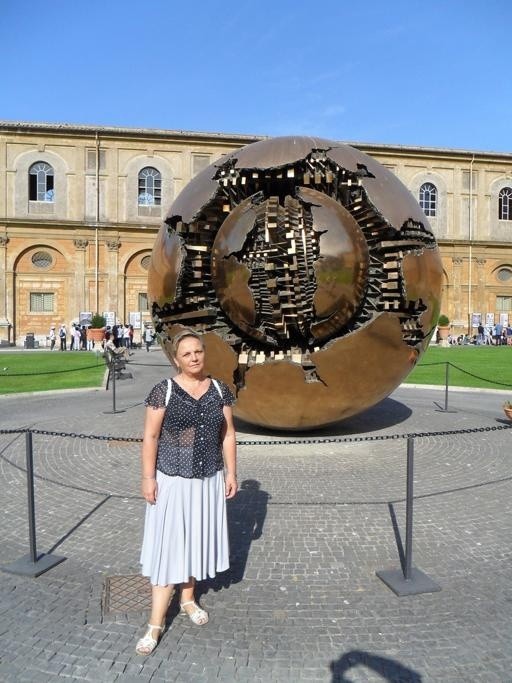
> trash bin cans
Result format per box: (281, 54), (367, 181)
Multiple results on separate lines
(26, 333), (34, 349)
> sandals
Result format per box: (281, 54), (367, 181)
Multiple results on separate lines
(136, 623), (166, 655)
(179, 601), (209, 625)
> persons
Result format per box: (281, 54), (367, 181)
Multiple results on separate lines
(134, 330), (237, 657)
(49, 323), (157, 352)
(448, 322), (512, 346)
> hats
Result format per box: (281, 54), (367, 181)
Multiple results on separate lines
(61, 325), (65, 327)
(51, 327), (55, 330)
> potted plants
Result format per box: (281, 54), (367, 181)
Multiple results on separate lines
(86, 313), (107, 341)
(437, 315), (450, 340)
(504, 399), (512, 421)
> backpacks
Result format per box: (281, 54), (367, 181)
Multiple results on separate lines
(60, 329), (65, 337)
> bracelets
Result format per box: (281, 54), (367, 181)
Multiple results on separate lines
(143, 476), (156, 480)
